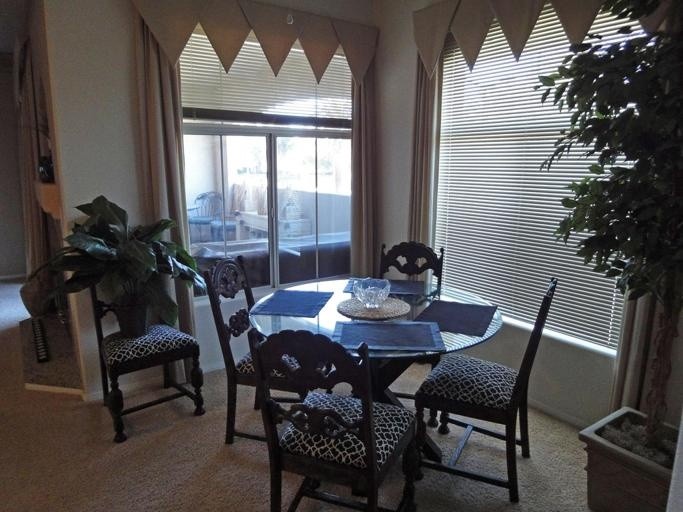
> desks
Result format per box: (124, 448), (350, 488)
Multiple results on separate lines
(234, 209), (312, 240)
(248, 275), (504, 462)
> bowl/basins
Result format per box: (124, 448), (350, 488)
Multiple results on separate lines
(354, 279), (390, 309)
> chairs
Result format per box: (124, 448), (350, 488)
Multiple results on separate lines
(211, 185), (246, 241)
(248, 327), (415, 512)
(378, 240), (444, 428)
(189, 190), (222, 244)
(87, 283), (205, 443)
(201, 254), (308, 445)
(412, 276), (559, 503)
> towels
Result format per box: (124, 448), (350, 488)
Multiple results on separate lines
(250, 289), (334, 318)
(343, 276), (425, 296)
(414, 300), (499, 337)
(332, 320), (447, 354)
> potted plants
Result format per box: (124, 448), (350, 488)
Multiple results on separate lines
(27, 195), (207, 338)
(534, 0), (682, 512)
